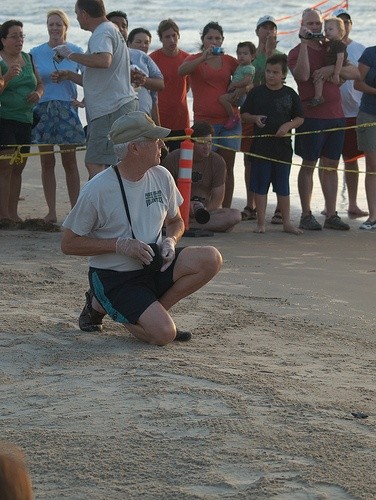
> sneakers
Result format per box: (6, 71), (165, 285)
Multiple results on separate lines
(359, 220), (376, 229)
(299, 214), (322, 230)
(323, 215), (350, 230)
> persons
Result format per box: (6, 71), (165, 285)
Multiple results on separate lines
(0, 0), (376, 236)
(61, 110), (223, 347)
(0, 443), (33, 500)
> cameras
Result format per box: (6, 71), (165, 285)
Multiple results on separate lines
(192, 196), (210, 224)
(213, 47), (225, 56)
(144, 243), (163, 274)
(307, 32), (323, 38)
(53, 52), (64, 63)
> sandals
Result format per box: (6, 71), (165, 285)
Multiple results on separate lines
(271, 208), (283, 224)
(240, 207), (257, 220)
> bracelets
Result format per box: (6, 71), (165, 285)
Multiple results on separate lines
(35, 91), (42, 100)
(141, 74), (146, 86)
(290, 120), (295, 128)
(0, 77), (8, 89)
(246, 86), (250, 93)
(66, 52), (74, 62)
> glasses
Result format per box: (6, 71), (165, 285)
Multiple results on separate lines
(132, 138), (160, 143)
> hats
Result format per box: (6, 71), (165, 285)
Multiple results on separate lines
(108, 111), (172, 144)
(333, 9), (352, 19)
(257, 16), (277, 29)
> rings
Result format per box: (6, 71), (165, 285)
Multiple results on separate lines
(133, 74), (137, 79)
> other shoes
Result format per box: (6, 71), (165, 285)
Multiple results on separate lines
(307, 97), (324, 108)
(224, 115), (240, 130)
(79, 289), (105, 332)
(175, 330), (192, 341)
